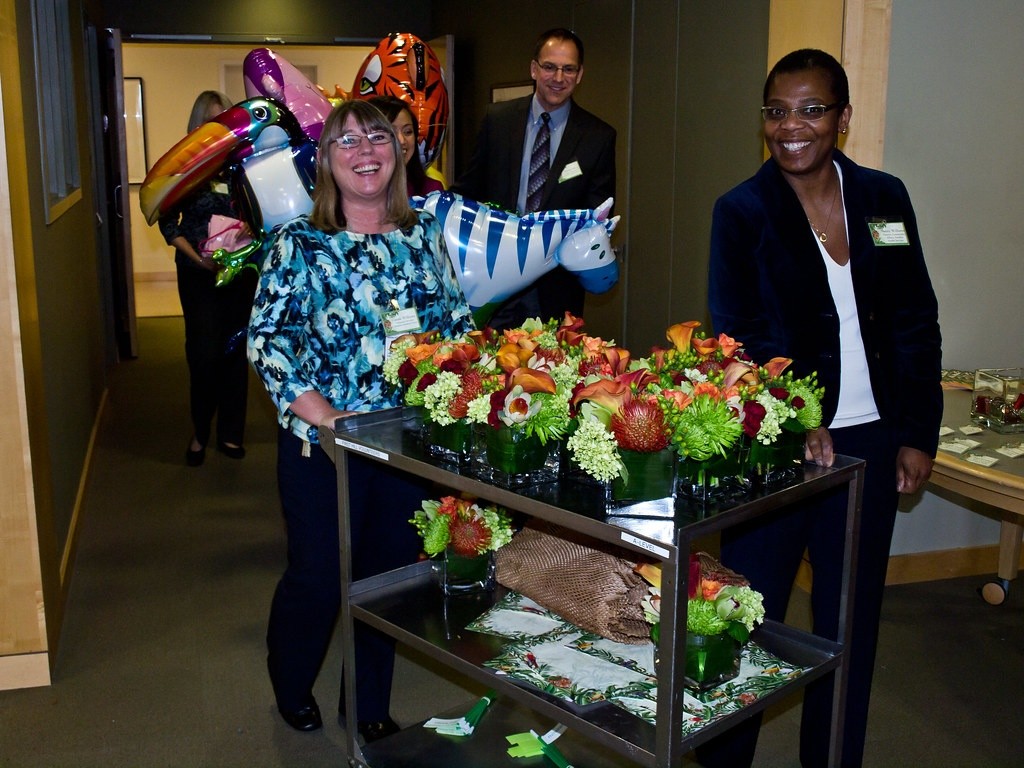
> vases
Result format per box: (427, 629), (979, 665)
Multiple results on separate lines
(428, 550), (488, 592)
(489, 414), (549, 472)
(683, 633), (742, 689)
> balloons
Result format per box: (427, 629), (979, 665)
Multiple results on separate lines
(139, 34), (449, 287)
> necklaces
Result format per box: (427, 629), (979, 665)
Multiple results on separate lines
(798, 177), (839, 242)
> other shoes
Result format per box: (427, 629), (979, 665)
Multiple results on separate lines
(216, 440), (245, 459)
(187, 436), (205, 466)
(339, 707), (401, 744)
(267, 654), (322, 732)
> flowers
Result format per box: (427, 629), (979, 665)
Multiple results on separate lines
(637, 554), (766, 690)
(411, 498), (506, 591)
(395, 314), (822, 486)
(319, 402), (865, 768)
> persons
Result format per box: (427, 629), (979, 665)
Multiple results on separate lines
(246, 97), (478, 746)
(460, 28), (619, 332)
(364, 95), (445, 199)
(693, 45), (946, 768)
(158, 90), (262, 465)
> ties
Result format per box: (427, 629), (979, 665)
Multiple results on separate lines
(525, 113), (551, 216)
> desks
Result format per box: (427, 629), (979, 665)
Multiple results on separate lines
(928, 382), (1024, 579)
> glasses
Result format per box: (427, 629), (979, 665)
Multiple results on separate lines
(533, 58), (580, 75)
(328, 130), (394, 148)
(761, 100), (849, 123)
